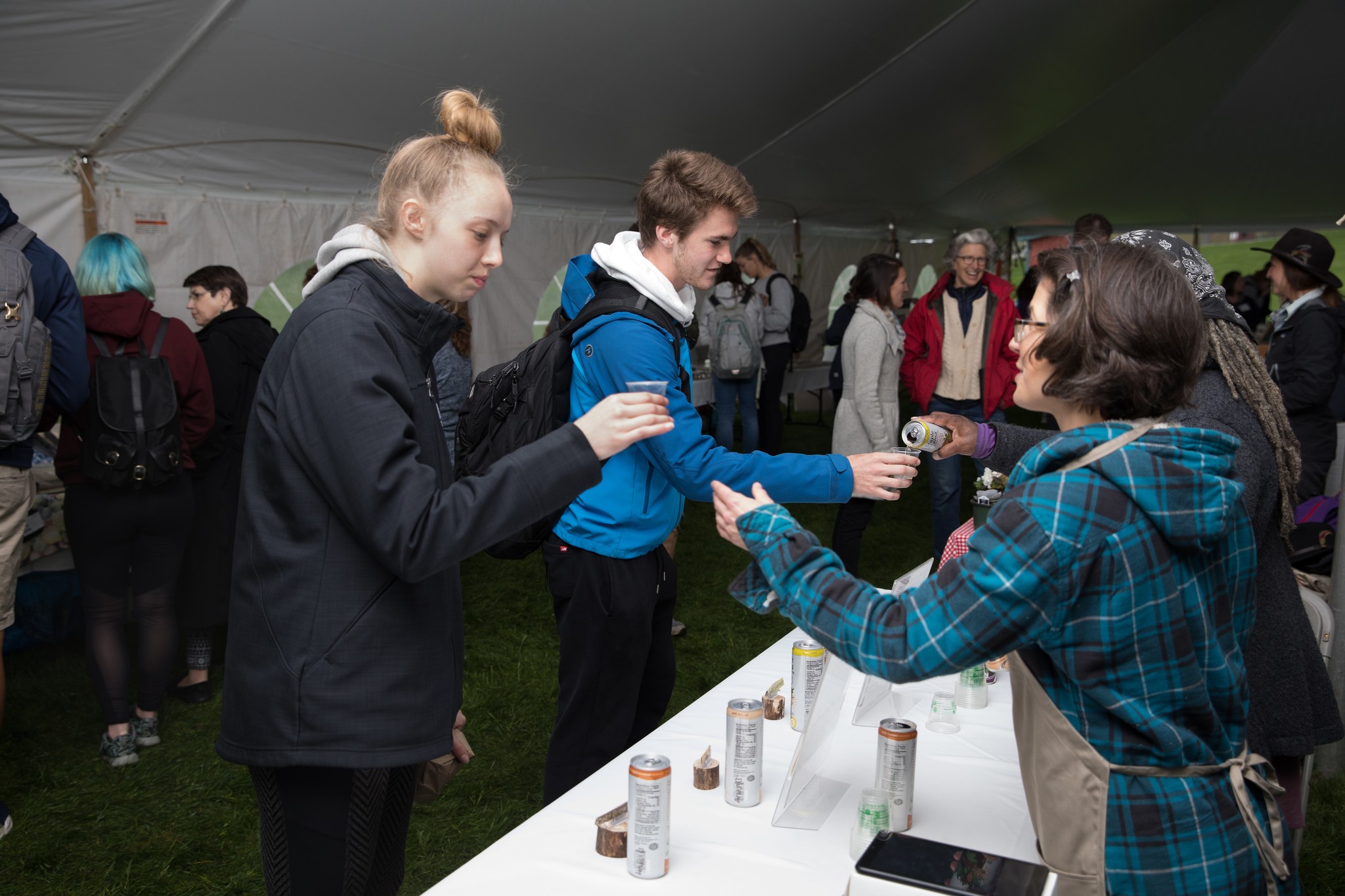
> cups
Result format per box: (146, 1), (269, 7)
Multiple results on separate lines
(924, 692), (960, 734)
(850, 788), (890, 862)
(627, 381), (668, 397)
(891, 447), (921, 479)
(955, 663), (988, 710)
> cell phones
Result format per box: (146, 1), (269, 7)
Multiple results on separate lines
(854, 830), (1049, 896)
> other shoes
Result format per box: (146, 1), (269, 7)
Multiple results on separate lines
(0, 807), (14, 840)
(671, 617), (686, 636)
(166, 669), (211, 703)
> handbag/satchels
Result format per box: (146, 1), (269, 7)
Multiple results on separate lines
(1294, 495), (1338, 529)
(1288, 522), (1334, 577)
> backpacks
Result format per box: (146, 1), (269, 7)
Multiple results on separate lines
(0, 221), (52, 447)
(79, 317), (185, 487)
(454, 268), (689, 560)
(707, 290), (760, 379)
(749, 273), (813, 353)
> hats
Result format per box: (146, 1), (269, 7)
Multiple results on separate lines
(1249, 227), (1344, 288)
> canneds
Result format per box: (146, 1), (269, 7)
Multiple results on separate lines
(790, 640), (825, 733)
(874, 718), (917, 832)
(724, 698), (764, 808)
(902, 420), (953, 453)
(626, 753), (672, 880)
(984, 662), (996, 685)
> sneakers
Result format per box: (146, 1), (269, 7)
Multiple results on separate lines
(131, 707), (160, 745)
(99, 724), (138, 766)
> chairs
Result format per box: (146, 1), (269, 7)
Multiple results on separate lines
(1290, 586), (1337, 872)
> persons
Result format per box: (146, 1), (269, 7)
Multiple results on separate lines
(55, 232), (214, 770)
(540, 148), (921, 814)
(710, 238), (1301, 896)
(0, 191), (90, 840)
(669, 212), (1345, 637)
(912, 228), (1345, 896)
(176, 265), (280, 703)
(432, 299), (473, 471)
(215, 89), (674, 896)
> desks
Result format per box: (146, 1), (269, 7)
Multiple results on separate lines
(421, 548), (1057, 896)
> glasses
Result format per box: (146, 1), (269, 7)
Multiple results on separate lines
(1013, 318), (1047, 343)
(957, 256), (989, 266)
(192, 289), (216, 302)
